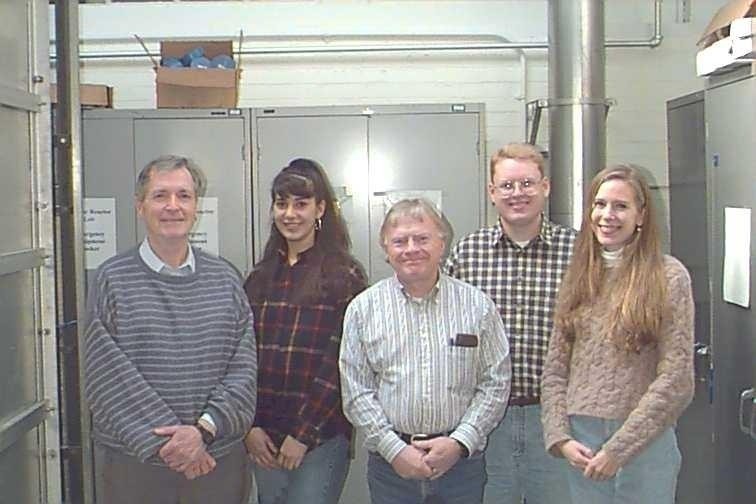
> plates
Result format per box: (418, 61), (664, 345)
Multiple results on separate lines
(82, 108), (253, 311)
(665, 73), (756, 504)
(250, 101), (488, 292)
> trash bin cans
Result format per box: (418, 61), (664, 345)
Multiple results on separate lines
(193, 420), (214, 444)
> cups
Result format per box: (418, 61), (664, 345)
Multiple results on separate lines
(496, 178), (541, 199)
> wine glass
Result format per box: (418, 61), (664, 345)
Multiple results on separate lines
(431, 468), (438, 475)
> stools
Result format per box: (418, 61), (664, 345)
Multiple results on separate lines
(508, 399), (541, 406)
(391, 431), (455, 445)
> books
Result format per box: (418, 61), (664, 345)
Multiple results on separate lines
(696, 1), (755, 45)
(134, 30), (243, 109)
(50, 83), (114, 111)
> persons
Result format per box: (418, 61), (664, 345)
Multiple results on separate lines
(336, 195), (515, 504)
(443, 142), (580, 504)
(237, 155), (368, 504)
(83, 153), (257, 502)
(537, 161), (696, 503)
